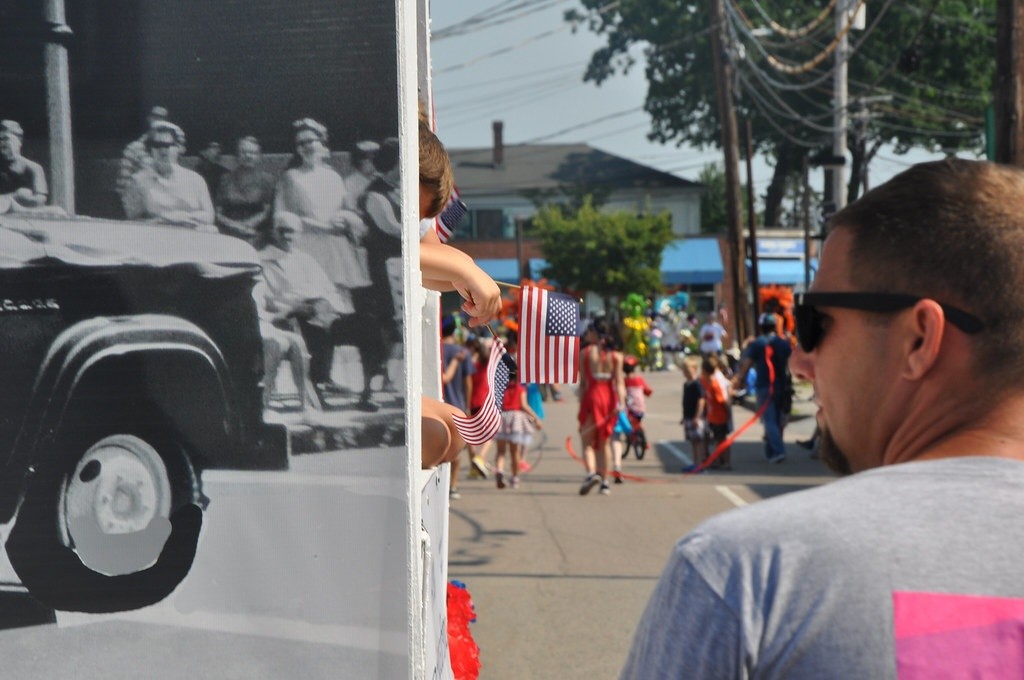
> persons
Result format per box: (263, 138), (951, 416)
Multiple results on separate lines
(415, 122), (503, 469)
(616, 159), (1024, 680)
(434, 299), (831, 496)
(0, 101), (404, 432)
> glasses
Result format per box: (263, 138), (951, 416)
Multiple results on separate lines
(791, 292), (981, 354)
(151, 140), (174, 149)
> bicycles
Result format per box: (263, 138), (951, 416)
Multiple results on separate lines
(618, 411), (647, 460)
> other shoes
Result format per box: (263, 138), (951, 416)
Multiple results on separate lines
(600, 484), (609, 494)
(796, 440), (814, 450)
(580, 474), (602, 495)
(466, 455), (489, 480)
(509, 477), (520, 488)
(495, 470), (505, 488)
(768, 454), (784, 465)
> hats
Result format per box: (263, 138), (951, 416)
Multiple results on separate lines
(758, 312), (775, 326)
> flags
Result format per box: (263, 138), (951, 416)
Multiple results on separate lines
(436, 181), (469, 244)
(517, 285), (581, 385)
(449, 337), (518, 445)
